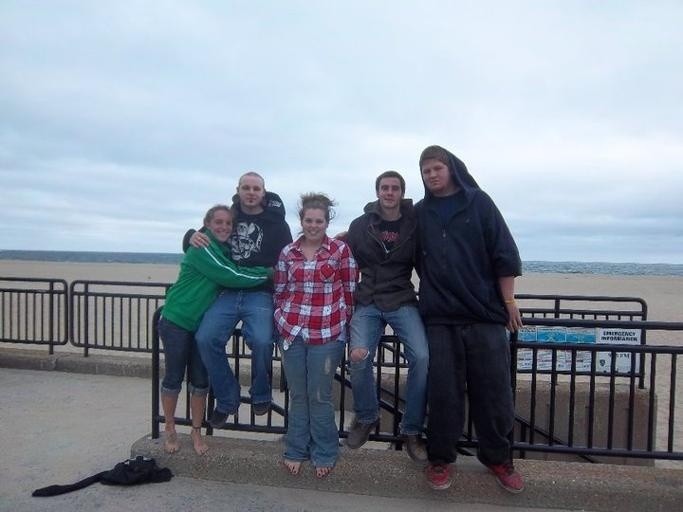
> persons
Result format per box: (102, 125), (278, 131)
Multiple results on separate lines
(333, 144), (526, 496)
(156, 204), (277, 456)
(272, 191), (359, 479)
(181, 171), (294, 430)
(345, 169), (434, 462)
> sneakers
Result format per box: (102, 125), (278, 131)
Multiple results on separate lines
(254, 401), (270, 416)
(348, 417), (380, 449)
(209, 407), (236, 428)
(484, 460), (524, 494)
(405, 432), (429, 466)
(424, 459), (454, 490)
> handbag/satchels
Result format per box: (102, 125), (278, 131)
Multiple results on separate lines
(101, 456), (159, 485)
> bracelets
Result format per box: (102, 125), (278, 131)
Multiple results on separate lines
(505, 299), (516, 305)
